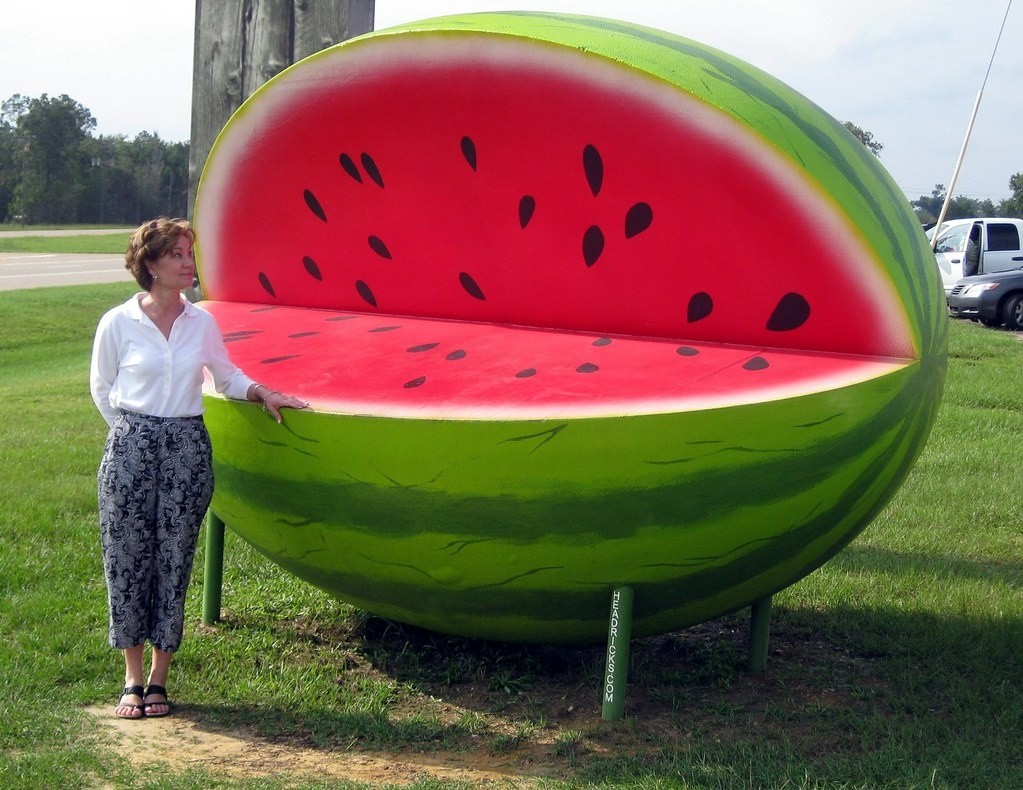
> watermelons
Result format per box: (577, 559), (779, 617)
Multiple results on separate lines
(191, 8), (946, 641)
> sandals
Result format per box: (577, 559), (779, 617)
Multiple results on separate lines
(115, 685), (145, 720)
(143, 684), (169, 717)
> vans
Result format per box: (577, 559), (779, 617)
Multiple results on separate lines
(923, 217), (1022, 301)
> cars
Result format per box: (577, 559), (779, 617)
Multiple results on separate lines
(948, 266), (1023, 331)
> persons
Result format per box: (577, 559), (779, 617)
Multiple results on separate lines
(958, 229), (977, 275)
(89, 219), (308, 720)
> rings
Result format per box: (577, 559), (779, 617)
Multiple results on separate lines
(289, 396), (295, 402)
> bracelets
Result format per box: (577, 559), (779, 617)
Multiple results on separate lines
(254, 384), (266, 402)
(262, 390), (280, 412)
